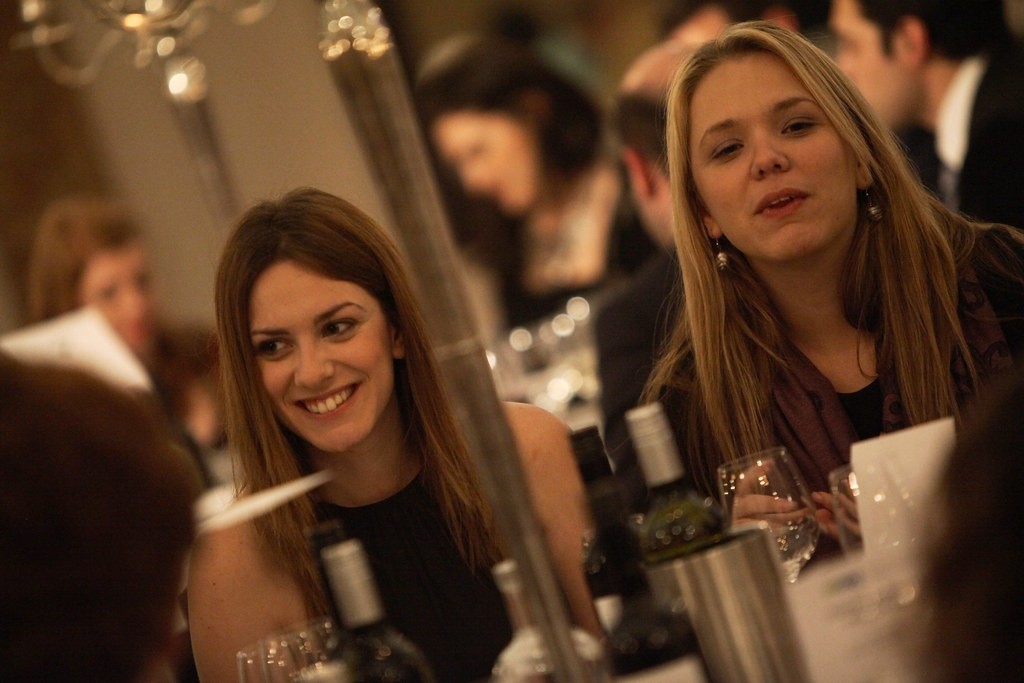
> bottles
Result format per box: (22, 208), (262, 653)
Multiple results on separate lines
(619, 394), (735, 617)
(320, 541), (436, 683)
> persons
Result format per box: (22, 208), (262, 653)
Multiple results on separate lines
(627, 18), (1023, 583)
(0, 343), (198, 683)
(182, 186), (610, 683)
(408, 0), (1024, 520)
(21, 184), (233, 454)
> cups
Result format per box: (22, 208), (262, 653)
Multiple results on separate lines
(260, 616), (355, 683)
(830, 464), (924, 575)
(718, 450), (819, 584)
(235, 635), (312, 682)
(666, 523), (810, 683)
